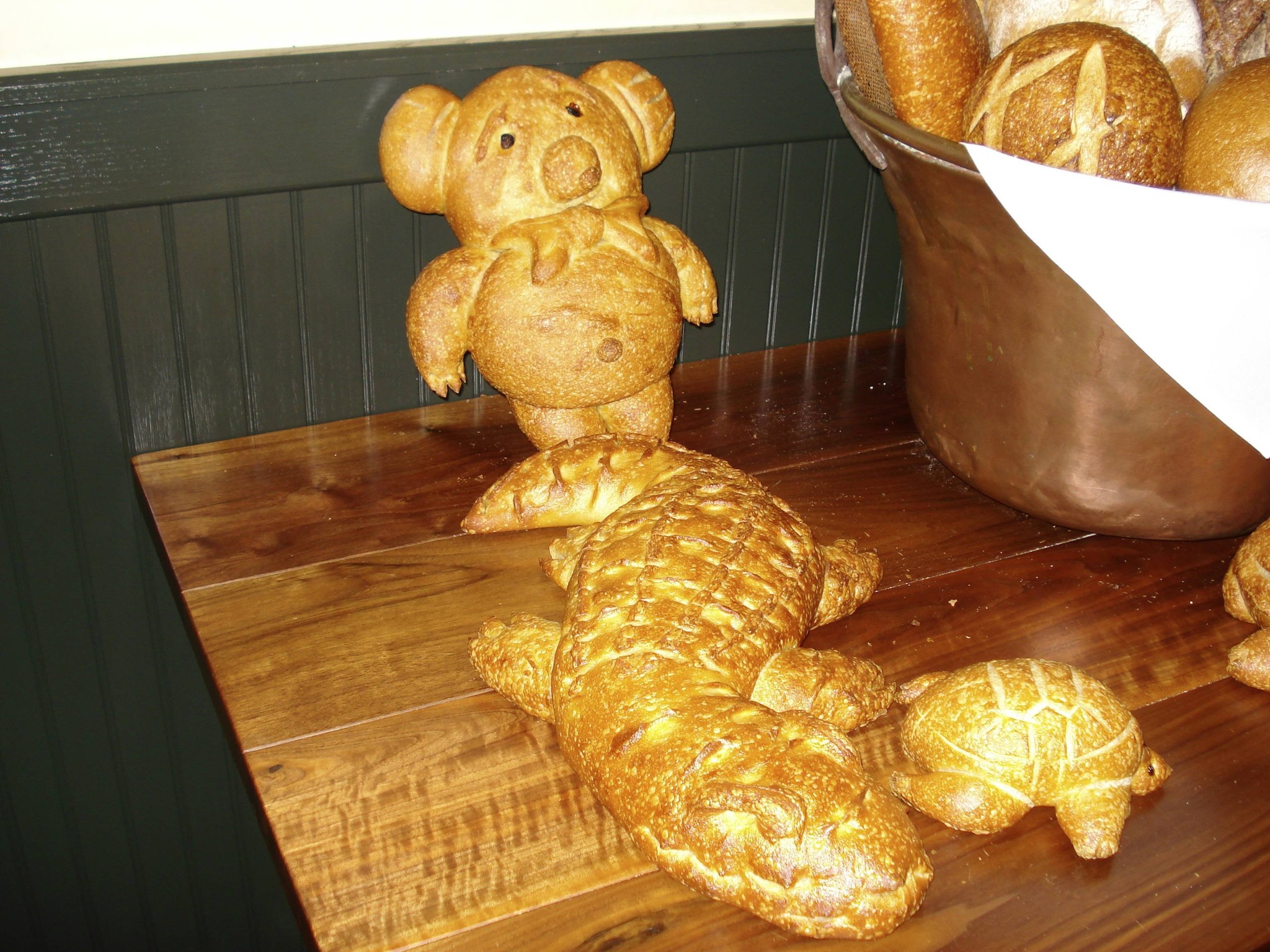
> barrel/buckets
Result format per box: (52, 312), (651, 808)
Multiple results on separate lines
(814, 0), (1270, 542)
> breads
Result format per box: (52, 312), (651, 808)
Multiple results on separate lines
(380, 61), (720, 449)
(461, 432), (1176, 938)
(1221, 516), (1270, 692)
(864, 0), (1270, 203)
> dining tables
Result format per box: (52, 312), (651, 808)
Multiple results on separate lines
(128, 328), (1270, 952)
